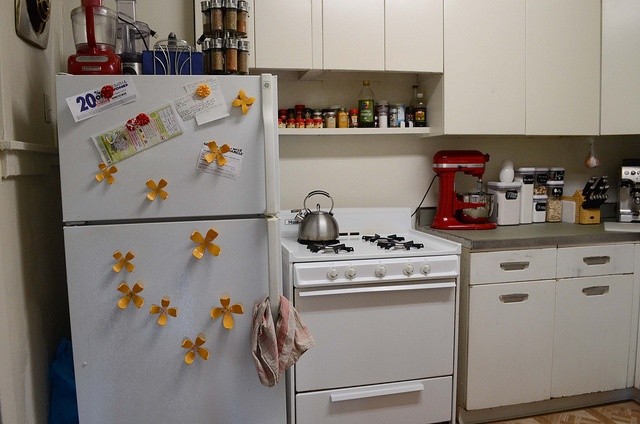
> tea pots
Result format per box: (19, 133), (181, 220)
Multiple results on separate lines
(290, 190), (340, 244)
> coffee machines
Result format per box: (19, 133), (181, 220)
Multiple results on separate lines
(619, 166), (639, 222)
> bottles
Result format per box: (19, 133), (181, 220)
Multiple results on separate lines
(281, 115), (286, 128)
(414, 93), (427, 126)
(305, 112), (314, 128)
(210, 0), (224, 31)
(338, 106), (348, 128)
(209, 38), (223, 70)
(239, 40), (249, 73)
(389, 108), (398, 127)
(533, 196), (548, 222)
(547, 181), (564, 222)
(358, 80), (375, 127)
(278, 104), (338, 114)
(550, 167), (565, 181)
(200, 1), (210, 37)
(225, 38), (238, 71)
(224, 0), (238, 31)
(202, 40), (211, 70)
(238, 0), (248, 34)
(377, 104), (389, 128)
(350, 109), (358, 127)
(410, 85), (420, 106)
(534, 167), (549, 195)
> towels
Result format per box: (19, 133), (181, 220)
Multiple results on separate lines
(250, 295), (314, 388)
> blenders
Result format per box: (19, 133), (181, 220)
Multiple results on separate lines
(431, 150), (498, 230)
(67, 0), (121, 74)
(114, 0), (151, 75)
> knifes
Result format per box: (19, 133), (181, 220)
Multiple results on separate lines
(581, 176), (609, 210)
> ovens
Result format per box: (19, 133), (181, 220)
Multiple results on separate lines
(292, 255), (460, 424)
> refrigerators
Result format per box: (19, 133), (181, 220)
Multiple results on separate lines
(52, 75), (286, 424)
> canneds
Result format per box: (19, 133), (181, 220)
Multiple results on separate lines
(305, 108), (314, 128)
(389, 104), (398, 127)
(330, 105), (341, 127)
(374, 100), (390, 128)
(349, 108), (360, 127)
(322, 109), (328, 127)
(287, 109), (295, 114)
(279, 109), (288, 114)
(295, 104), (305, 111)
(278, 113), (287, 128)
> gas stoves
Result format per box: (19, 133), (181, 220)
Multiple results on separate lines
(280, 228), (462, 256)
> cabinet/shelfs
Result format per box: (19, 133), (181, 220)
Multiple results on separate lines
(384, 0), (444, 73)
(524, 1), (602, 136)
(422, 0), (526, 136)
(323, 0), (385, 71)
(601, 1), (639, 136)
(458, 246), (558, 423)
(278, 125), (431, 137)
(255, 0), (312, 71)
(557, 241), (640, 412)
